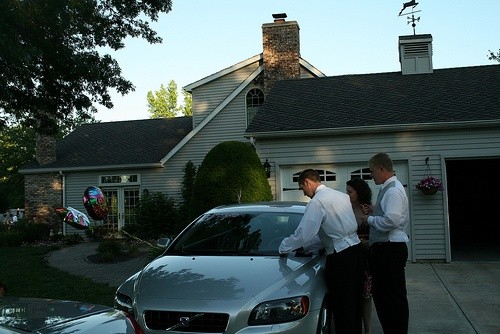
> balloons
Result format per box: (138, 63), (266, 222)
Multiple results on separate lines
(55, 207), (90, 230)
(82, 187), (109, 220)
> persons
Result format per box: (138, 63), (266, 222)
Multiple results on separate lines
(13, 215), (18, 228)
(346, 179), (374, 334)
(279, 169), (365, 334)
(360, 152), (409, 334)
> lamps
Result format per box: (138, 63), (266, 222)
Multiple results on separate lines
(263, 158), (272, 178)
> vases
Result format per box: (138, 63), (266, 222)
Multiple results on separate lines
(421, 186), (437, 194)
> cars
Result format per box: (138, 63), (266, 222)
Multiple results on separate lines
(0, 208), (24, 226)
(0, 295), (144, 334)
(112, 201), (333, 334)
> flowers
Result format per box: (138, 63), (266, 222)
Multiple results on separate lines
(412, 177), (445, 194)
